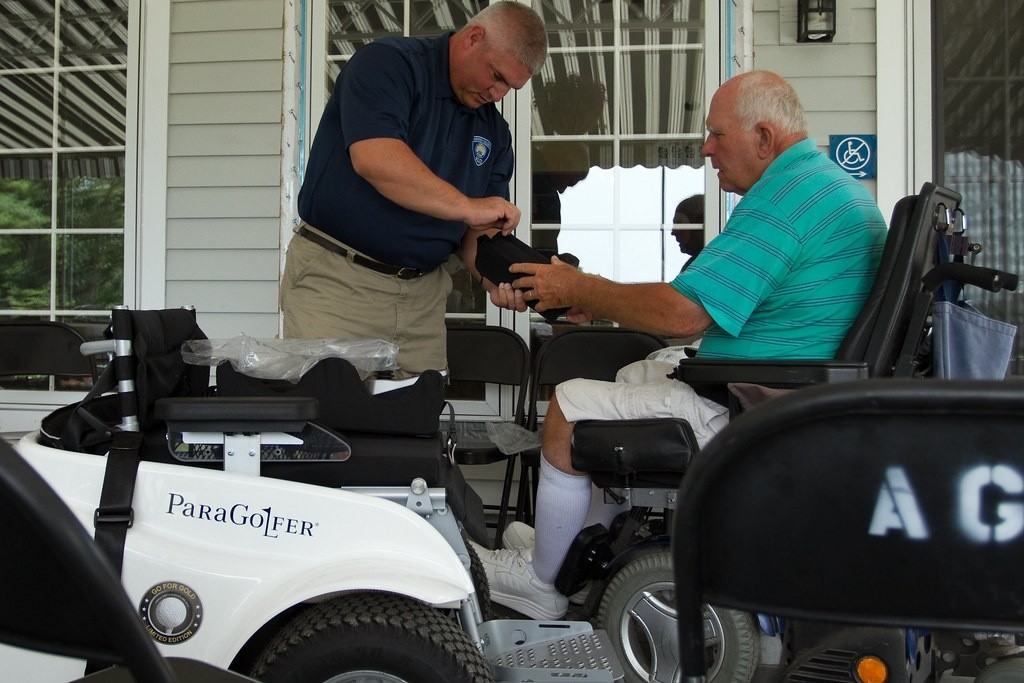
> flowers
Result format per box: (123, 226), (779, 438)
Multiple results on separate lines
(534, 71), (608, 109)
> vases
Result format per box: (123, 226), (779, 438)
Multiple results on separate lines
(537, 104), (597, 134)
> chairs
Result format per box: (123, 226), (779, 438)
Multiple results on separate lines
(514, 330), (668, 535)
(1, 323), (96, 390)
(443, 326), (525, 549)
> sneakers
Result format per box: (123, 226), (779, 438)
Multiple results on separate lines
(503, 521), (592, 602)
(469, 539), (567, 620)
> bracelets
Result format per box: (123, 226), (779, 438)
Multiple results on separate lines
(478, 274), (484, 288)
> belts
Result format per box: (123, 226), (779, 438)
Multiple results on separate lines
(297, 226), (434, 280)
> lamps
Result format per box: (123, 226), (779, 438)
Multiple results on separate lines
(796, 0), (837, 43)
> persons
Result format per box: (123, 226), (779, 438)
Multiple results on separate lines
(464, 69), (890, 620)
(280, 0), (549, 387)
(671, 194), (705, 273)
(531, 141), (590, 258)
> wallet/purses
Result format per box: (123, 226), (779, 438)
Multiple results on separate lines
(475, 231), (571, 321)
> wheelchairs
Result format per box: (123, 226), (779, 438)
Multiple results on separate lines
(482, 182), (1018, 683)
